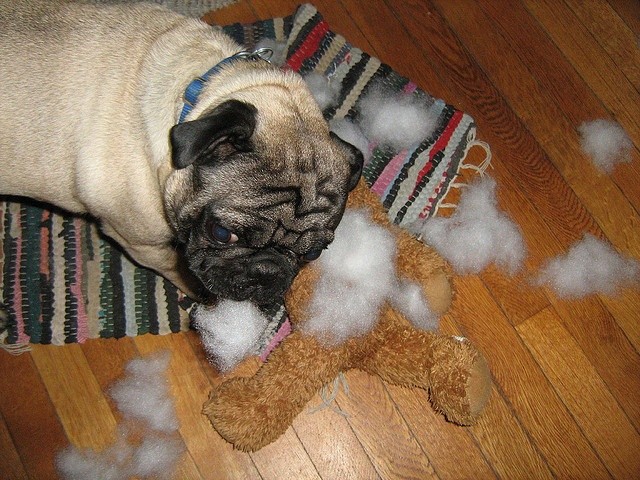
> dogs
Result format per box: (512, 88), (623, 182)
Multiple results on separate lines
(0, 0), (366, 311)
